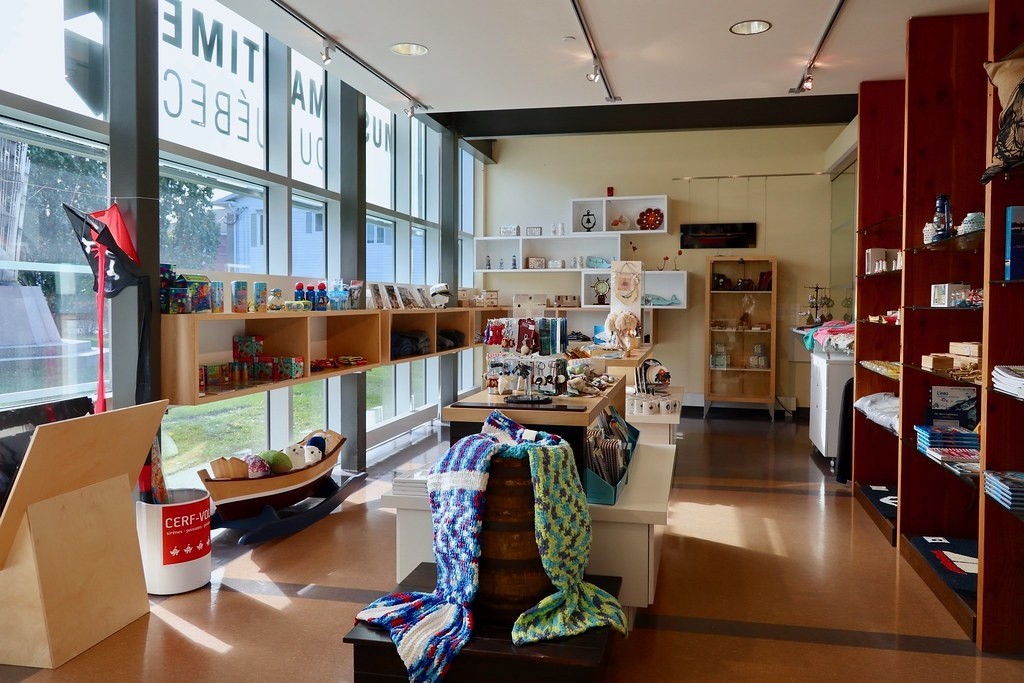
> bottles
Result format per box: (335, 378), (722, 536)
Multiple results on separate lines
(511, 254), (517, 269)
(485, 256), (491, 269)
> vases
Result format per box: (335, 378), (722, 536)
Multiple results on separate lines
(615, 335), (633, 357)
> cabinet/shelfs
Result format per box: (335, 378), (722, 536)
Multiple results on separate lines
(382, 342), (684, 630)
(473, 196), (689, 345)
(706, 255), (778, 422)
(853, 1), (1024, 655)
(160, 306), (472, 406)
(809, 353), (854, 467)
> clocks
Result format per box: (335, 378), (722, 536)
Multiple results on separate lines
(591, 277), (611, 304)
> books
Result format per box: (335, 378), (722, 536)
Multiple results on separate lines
(914, 364), (1024, 511)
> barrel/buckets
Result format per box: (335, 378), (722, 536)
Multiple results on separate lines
(135, 485), (214, 595)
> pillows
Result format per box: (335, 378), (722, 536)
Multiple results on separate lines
(980, 57), (1024, 184)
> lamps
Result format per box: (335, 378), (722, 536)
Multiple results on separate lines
(320, 39), (336, 65)
(404, 99), (417, 118)
(585, 58), (600, 83)
(802, 66), (812, 92)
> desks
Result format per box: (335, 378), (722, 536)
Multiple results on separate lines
(343, 562), (621, 683)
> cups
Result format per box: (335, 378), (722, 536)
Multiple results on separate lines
(625, 393), (681, 415)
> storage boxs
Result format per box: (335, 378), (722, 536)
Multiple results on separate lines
(922, 341), (982, 371)
(601, 415), (641, 469)
(865, 248), (898, 274)
(234, 335), (304, 381)
(460, 299), (469, 308)
(930, 283), (971, 307)
(928, 387), (978, 429)
(585, 462), (628, 504)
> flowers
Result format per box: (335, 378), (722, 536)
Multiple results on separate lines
(604, 311), (638, 350)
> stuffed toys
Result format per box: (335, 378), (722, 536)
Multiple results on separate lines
(567, 364), (614, 398)
(242, 435), (327, 480)
